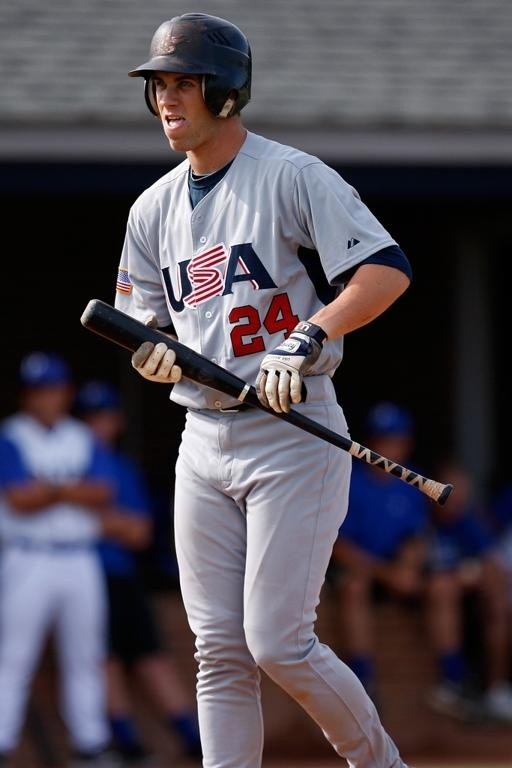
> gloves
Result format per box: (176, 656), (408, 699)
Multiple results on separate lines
(130, 311), (185, 387)
(253, 319), (329, 415)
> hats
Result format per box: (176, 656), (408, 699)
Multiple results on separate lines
(19, 353), (123, 418)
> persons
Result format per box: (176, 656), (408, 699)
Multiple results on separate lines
(1, 350), (132, 768)
(111, 9), (416, 768)
(415, 453), (511, 724)
(63, 381), (204, 768)
(329, 402), (485, 706)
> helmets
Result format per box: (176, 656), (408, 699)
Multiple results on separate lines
(128, 12), (253, 121)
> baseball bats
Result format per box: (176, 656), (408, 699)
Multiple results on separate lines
(80, 298), (452, 505)
